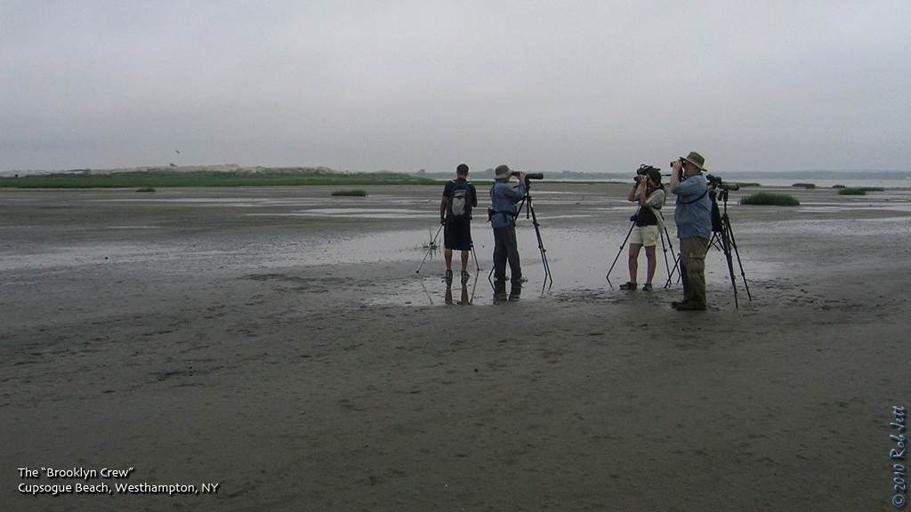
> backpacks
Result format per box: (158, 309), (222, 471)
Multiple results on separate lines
(448, 179), (473, 218)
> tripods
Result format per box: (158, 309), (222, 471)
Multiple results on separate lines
(487, 188), (553, 281)
(416, 219), (480, 273)
(606, 210), (681, 284)
(664, 201), (752, 309)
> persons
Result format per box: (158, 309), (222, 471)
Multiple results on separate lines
(490, 164), (530, 282)
(619, 166), (667, 292)
(444, 280), (473, 304)
(439, 164), (479, 281)
(491, 282), (523, 306)
(667, 151), (714, 311)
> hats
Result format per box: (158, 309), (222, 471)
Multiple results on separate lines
(678, 152), (708, 171)
(493, 165), (512, 179)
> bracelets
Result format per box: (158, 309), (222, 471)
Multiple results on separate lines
(633, 185), (639, 189)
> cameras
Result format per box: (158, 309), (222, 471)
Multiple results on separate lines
(633, 168), (649, 184)
(669, 160), (686, 167)
(527, 172), (544, 179)
(718, 183), (740, 191)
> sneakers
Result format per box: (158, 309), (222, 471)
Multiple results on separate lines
(445, 270), (469, 277)
(672, 301), (706, 310)
(496, 276), (526, 281)
(619, 282), (637, 288)
(643, 284), (652, 291)
